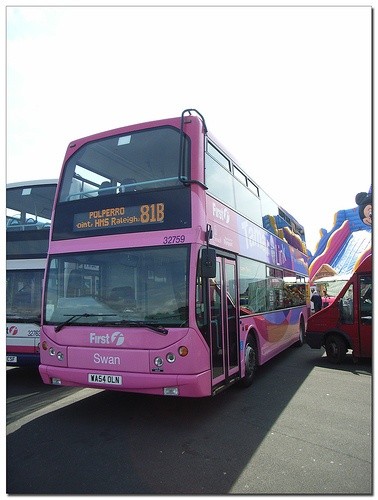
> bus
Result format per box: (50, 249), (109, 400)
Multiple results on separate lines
(39, 108), (310, 398)
(5, 180), (102, 367)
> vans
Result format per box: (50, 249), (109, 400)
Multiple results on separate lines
(306, 252), (371, 363)
